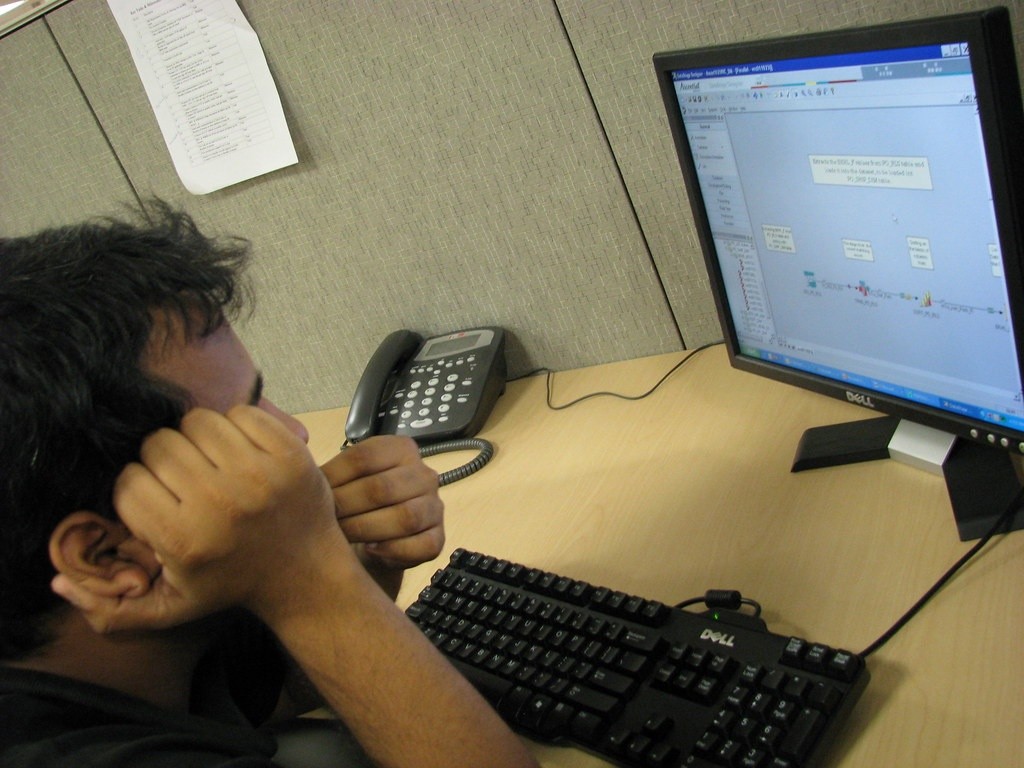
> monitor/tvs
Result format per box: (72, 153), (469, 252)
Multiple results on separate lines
(650, 6), (1024, 541)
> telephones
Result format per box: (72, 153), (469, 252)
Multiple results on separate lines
(343, 325), (515, 444)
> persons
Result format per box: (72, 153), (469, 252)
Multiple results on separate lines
(2, 195), (538, 768)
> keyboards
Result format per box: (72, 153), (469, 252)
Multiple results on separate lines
(405, 548), (869, 768)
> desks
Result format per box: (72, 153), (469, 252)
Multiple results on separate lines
(283, 338), (1024, 766)
